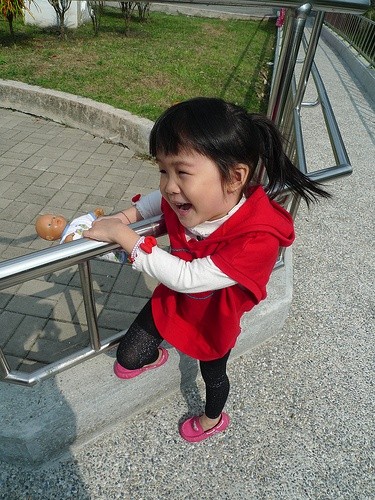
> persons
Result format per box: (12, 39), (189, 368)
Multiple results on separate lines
(83, 97), (333, 442)
(35, 208), (134, 263)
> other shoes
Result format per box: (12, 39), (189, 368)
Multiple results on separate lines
(113, 347), (169, 379)
(180, 411), (229, 441)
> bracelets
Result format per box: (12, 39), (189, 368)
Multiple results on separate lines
(122, 211), (132, 224)
(131, 236), (145, 259)
